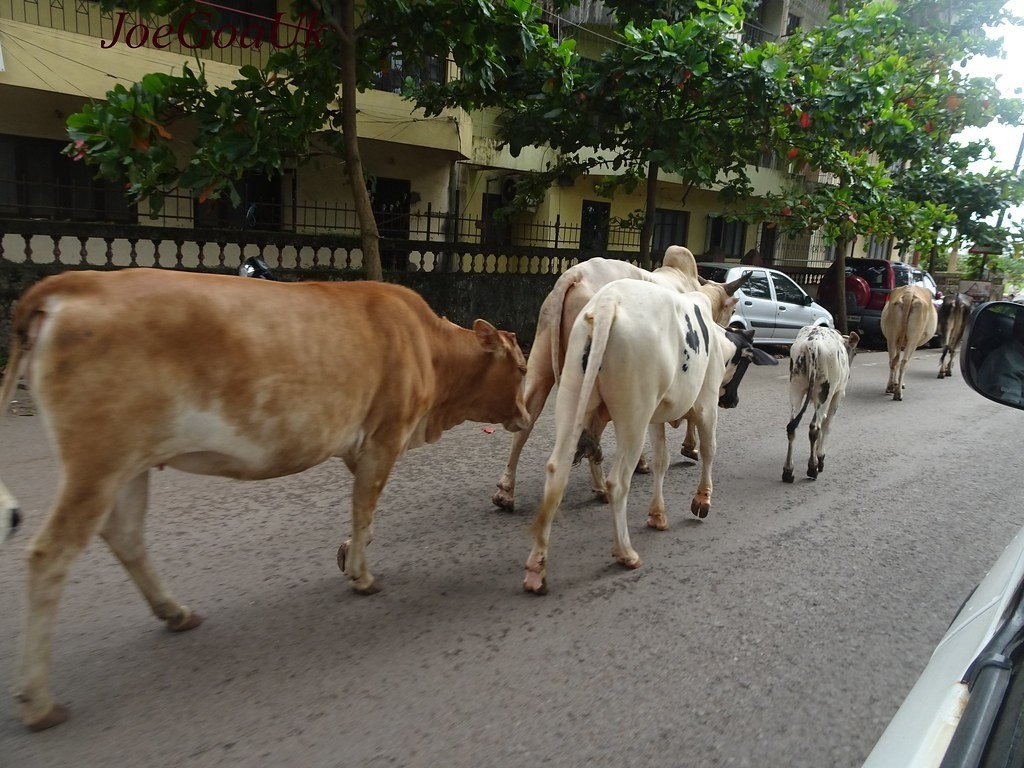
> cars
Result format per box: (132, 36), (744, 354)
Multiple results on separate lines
(939, 301), (1024, 768)
(697, 262), (834, 344)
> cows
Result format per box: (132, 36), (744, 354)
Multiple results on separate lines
(938, 291), (981, 379)
(782, 326), (861, 485)
(0, 268), (535, 733)
(491, 245), (780, 596)
(879, 283), (938, 400)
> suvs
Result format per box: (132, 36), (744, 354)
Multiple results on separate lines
(817, 257), (944, 348)
